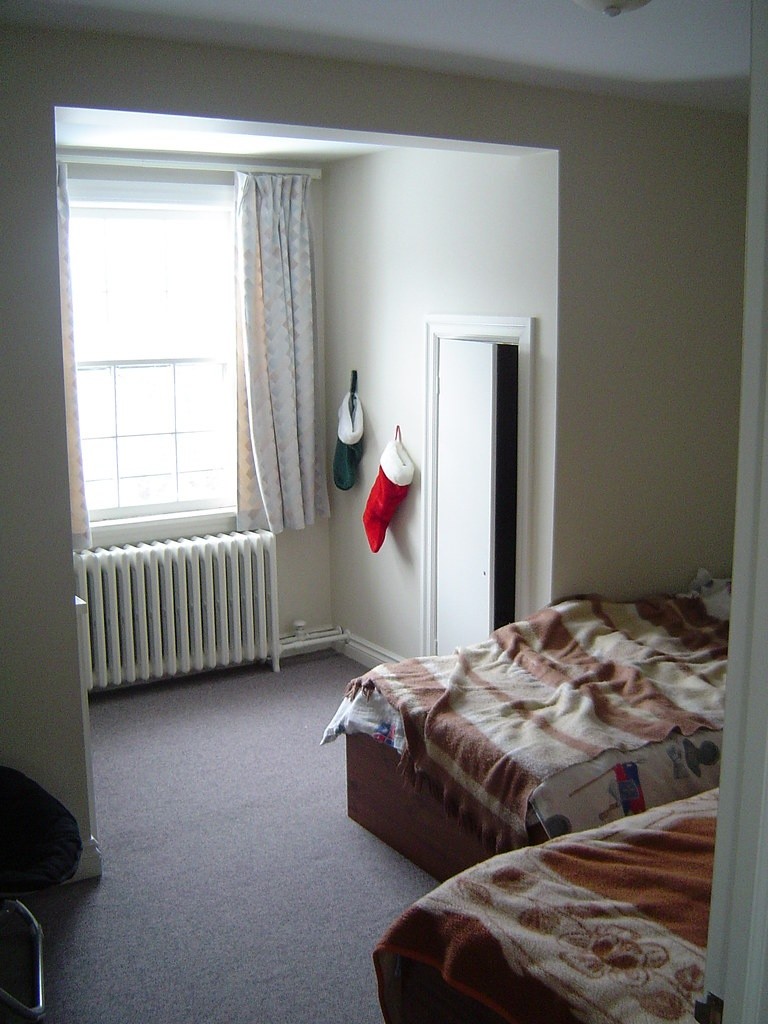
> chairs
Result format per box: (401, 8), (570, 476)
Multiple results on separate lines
(0, 765), (83, 1018)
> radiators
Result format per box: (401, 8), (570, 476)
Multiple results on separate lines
(73, 529), (282, 696)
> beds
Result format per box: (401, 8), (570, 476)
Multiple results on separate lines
(320, 574), (733, 885)
(372, 785), (720, 1024)
